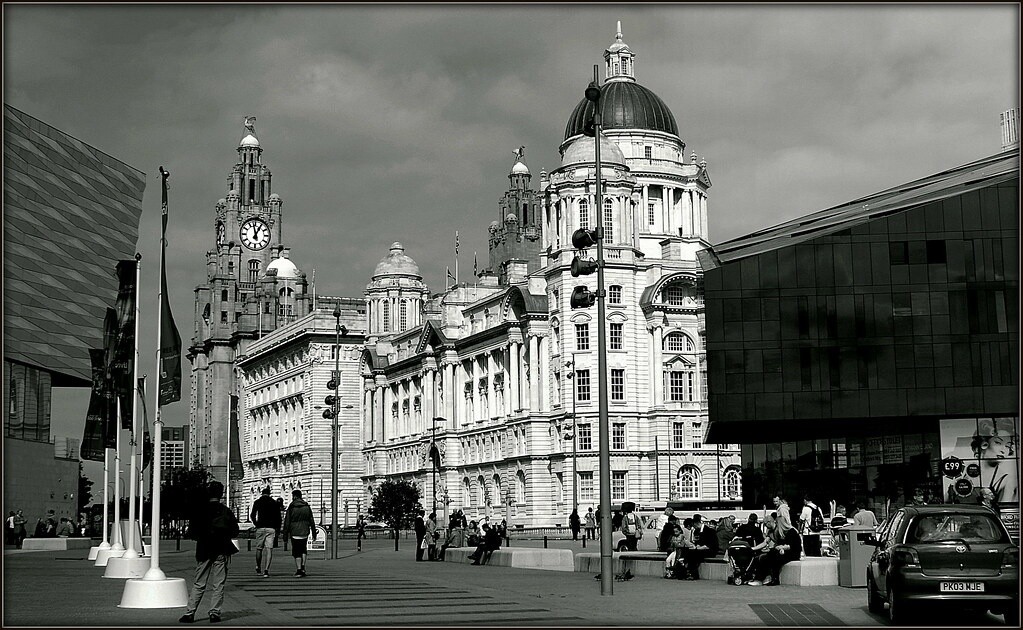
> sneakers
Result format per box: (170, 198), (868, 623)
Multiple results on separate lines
(293, 570), (305, 578)
(256, 566), (261, 573)
(263, 570), (269, 577)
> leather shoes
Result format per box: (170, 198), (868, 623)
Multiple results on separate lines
(210, 614), (221, 622)
(179, 614), (194, 623)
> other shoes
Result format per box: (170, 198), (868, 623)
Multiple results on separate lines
(471, 561), (480, 565)
(434, 557), (443, 561)
(686, 577), (699, 580)
(748, 575), (772, 586)
(665, 569), (674, 574)
(467, 555), (476, 560)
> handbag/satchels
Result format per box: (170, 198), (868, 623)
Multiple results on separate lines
(470, 535), (481, 547)
(635, 530), (641, 539)
(445, 540), (452, 543)
(421, 539), (428, 549)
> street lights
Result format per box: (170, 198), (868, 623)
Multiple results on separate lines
(568, 78), (615, 593)
(430, 416), (446, 526)
(94, 462), (140, 504)
(321, 300), (342, 560)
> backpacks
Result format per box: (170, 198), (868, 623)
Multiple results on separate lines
(805, 505), (823, 532)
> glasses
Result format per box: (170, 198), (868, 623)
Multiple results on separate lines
(764, 521), (770, 525)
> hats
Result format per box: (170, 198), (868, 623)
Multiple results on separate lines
(669, 514), (676, 521)
(749, 514), (757, 522)
(262, 488), (270, 494)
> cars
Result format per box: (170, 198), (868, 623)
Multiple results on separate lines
(321, 524), (333, 535)
(863, 505), (1020, 627)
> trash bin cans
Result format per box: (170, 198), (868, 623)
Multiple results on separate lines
(838, 526), (877, 589)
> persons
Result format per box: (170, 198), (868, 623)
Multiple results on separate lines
(356, 515), (367, 539)
(7, 509), (90, 550)
(595, 505), (602, 541)
(415, 510), (426, 561)
(980, 488), (1001, 520)
(250, 489), (281, 577)
(569, 509), (580, 540)
(178, 481), (239, 623)
(906, 488), (927, 507)
(283, 490), (317, 577)
(948, 419), (1018, 504)
(613, 496), (879, 586)
(584, 507), (595, 540)
(438, 509), (510, 565)
(425, 513), (438, 560)
